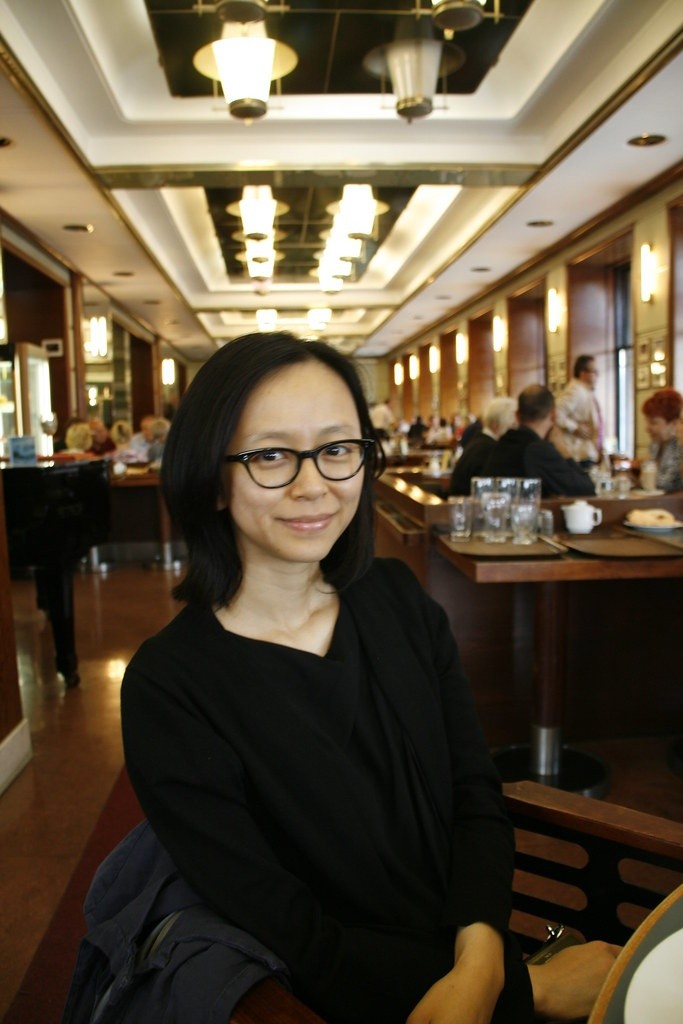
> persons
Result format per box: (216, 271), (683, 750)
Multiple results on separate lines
(548, 354), (606, 472)
(365, 386), (596, 496)
(59, 415), (172, 472)
(631, 388), (682, 493)
(120, 330), (629, 1023)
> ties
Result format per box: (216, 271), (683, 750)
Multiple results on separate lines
(592, 396), (604, 451)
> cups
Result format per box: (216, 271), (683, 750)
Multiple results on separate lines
(563, 500), (602, 535)
(448, 476), (553, 546)
(638, 461), (658, 492)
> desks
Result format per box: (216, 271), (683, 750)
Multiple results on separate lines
(0, 455), (110, 689)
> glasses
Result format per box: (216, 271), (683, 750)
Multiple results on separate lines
(223, 438), (374, 489)
(588, 367), (599, 377)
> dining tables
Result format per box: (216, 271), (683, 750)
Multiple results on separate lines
(430, 524), (683, 813)
(77, 469), (185, 570)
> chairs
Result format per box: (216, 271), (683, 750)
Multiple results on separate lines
(500, 781), (683, 1024)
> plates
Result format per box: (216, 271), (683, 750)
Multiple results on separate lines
(625, 521), (683, 533)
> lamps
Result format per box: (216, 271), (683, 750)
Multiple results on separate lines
(430, 0), (487, 31)
(225, 184), (391, 295)
(361, 0), (471, 117)
(192, 0), (299, 118)
